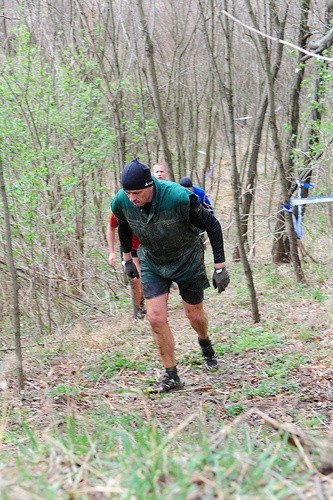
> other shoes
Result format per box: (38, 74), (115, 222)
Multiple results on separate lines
(198, 336), (219, 370)
(150, 375), (183, 393)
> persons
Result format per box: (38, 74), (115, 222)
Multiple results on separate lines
(181, 177), (213, 214)
(107, 213), (146, 319)
(154, 164), (168, 180)
(110, 159), (230, 393)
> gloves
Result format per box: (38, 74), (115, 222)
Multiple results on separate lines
(212, 267), (231, 293)
(124, 258), (139, 280)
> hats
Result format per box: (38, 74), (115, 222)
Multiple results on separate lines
(180, 177), (193, 187)
(122, 158), (154, 190)
